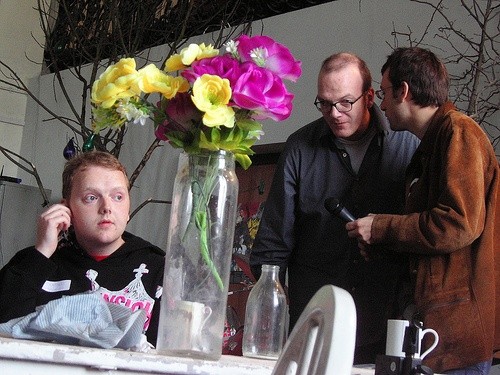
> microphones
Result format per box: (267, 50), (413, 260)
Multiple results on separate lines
(324, 197), (358, 224)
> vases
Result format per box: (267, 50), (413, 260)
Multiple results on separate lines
(156, 148), (239, 361)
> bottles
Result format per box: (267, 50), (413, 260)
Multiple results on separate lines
(242, 265), (287, 362)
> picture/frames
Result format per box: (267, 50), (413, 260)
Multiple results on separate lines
(229, 142), (286, 285)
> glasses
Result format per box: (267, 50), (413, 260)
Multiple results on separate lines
(375, 86), (396, 100)
(314, 94), (363, 114)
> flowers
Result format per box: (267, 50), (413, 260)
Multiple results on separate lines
(90, 34), (302, 293)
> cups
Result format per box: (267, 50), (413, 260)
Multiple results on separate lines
(173, 300), (212, 348)
(385, 319), (439, 361)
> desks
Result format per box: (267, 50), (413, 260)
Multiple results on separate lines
(0, 336), (376, 375)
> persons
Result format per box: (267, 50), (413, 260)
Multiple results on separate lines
(248, 52), (423, 365)
(347, 47), (500, 375)
(0, 152), (166, 349)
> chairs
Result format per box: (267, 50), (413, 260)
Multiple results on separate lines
(271, 285), (357, 375)
